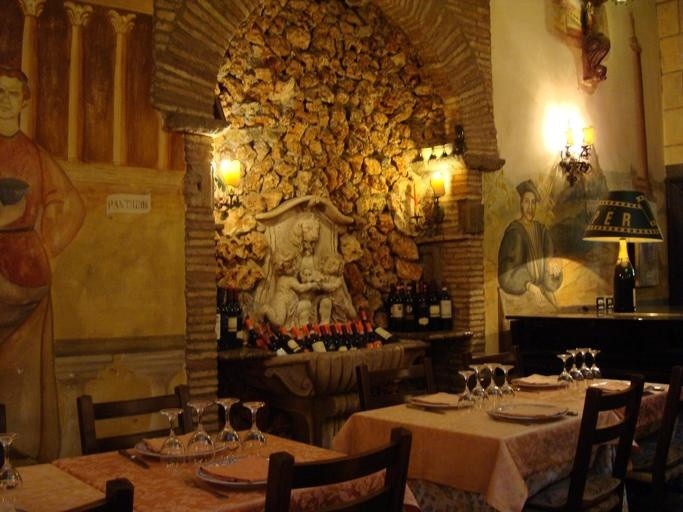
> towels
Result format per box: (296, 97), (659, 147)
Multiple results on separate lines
(414, 390), (460, 407)
(496, 403), (570, 420)
(515, 371), (561, 387)
(144, 430), (212, 456)
(595, 379), (629, 393)
(198, 456), (269, 482)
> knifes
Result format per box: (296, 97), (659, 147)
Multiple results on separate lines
(406, 404), (445, 414)
(118, 448), (151, 469)
(184, 481), (230, 501)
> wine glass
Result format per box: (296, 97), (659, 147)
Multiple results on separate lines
(0, 433), (24, 506)
(456, 363), (516, 418)
(557, 347), (603, 396)
(159, 399), (269, 479)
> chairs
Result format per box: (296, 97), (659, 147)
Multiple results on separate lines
(75, 383), (194, 455)
(623, 374), (683, 511)
(357, 356), (436, 410)
(265, 428), (412, 512)
(16, 477), (135, 512)
(459, 344), (522, 394)
(525, 374), (644, 511)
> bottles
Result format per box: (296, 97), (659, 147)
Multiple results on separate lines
(216, 291), (244, 352)
(385, 278), (453, 334)
(612, 238), (637, 314)
(242, 314), (383, 357)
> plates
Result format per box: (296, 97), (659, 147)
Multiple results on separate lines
(195, 457), (271, 489)
(511, 381), (566, 389)
(410, 399), (474, 409)
(486, 400), (570, 422)
(133, 442), (226, 461)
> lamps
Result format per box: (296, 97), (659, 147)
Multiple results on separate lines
(582, 190), (668, 314)
(427, 174), (450, 223)
(219, 157), (245, 207)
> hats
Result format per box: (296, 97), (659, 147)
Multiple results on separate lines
(516, 179), (543, 203)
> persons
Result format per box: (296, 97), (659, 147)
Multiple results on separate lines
(257, 252), (356, 328)
(498, 180), (564, 330)
(2, 65), (86, 467)
